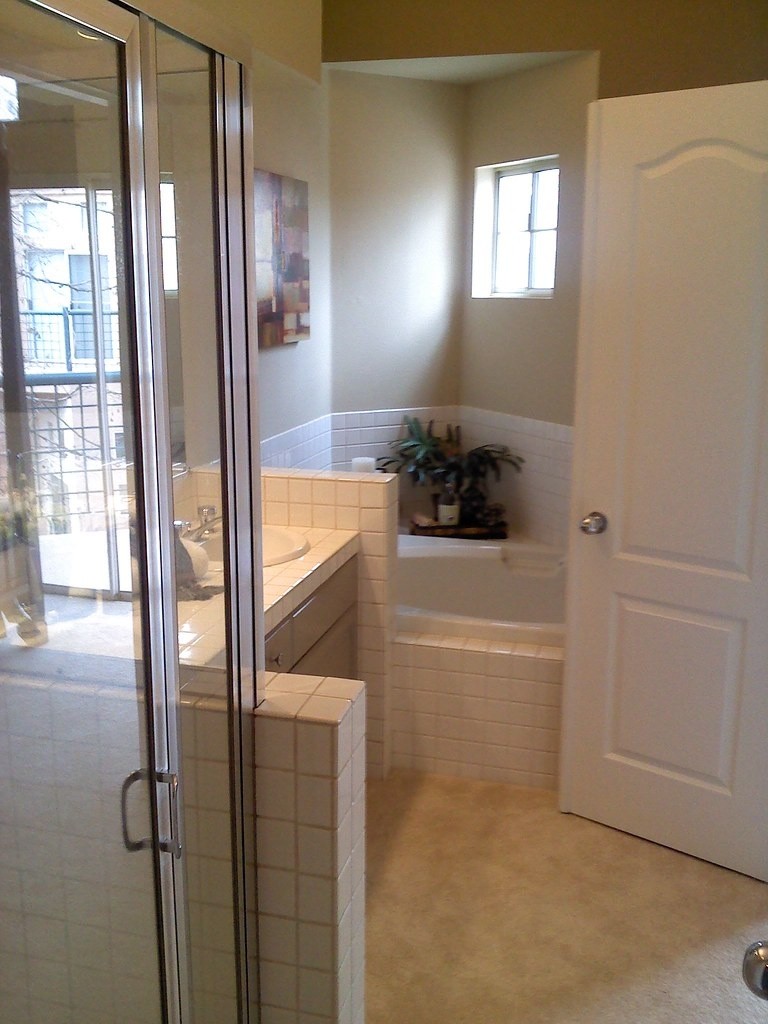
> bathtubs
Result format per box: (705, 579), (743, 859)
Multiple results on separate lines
(394, 533), (568, 791)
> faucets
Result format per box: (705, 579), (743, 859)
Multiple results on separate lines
(181, 515), (222, 542)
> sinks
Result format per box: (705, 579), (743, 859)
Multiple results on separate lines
(196, 527), (309, 571)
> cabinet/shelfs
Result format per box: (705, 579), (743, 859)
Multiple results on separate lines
(264, 554), (358, 681)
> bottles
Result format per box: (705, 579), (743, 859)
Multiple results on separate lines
(437, 482), (461, 526)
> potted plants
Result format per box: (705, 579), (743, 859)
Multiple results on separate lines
(374, 414), (525, 524)
